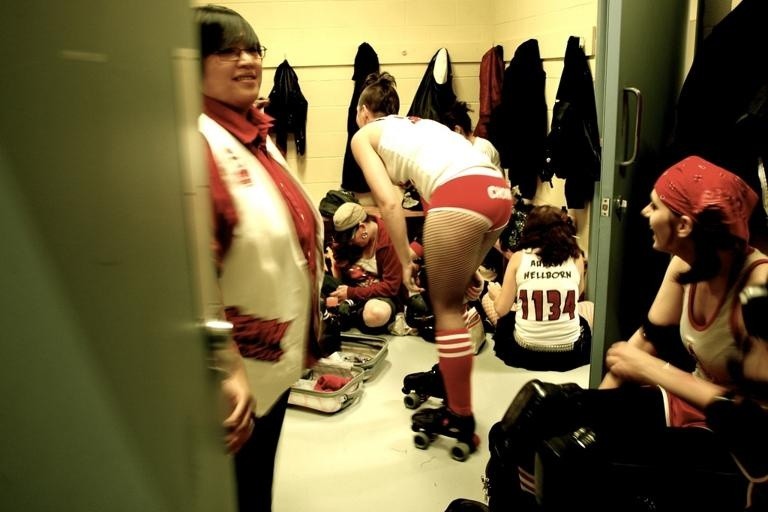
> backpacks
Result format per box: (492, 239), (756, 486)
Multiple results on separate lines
(481, 379), (599, 510)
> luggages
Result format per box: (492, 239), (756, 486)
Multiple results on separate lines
(287, 332), (388, 413)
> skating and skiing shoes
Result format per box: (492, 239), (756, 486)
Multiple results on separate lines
(402, 363), (448, 409)
(412, 406), (479, 461)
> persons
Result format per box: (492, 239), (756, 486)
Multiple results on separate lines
(347, 69), (517, 439)
(311, 191), (406, 328)
(404, 101), (500, 356)
(482, 205), (592, 373)
(193, 3), (329, 512)
(443, 154), (768, 511)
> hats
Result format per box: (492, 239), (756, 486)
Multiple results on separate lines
(333, 202), (367, 243)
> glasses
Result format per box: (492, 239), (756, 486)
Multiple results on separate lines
(218, 46), (267, 61)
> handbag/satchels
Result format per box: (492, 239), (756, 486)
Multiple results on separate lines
(319, 190), (360, 219)
(499, 193), (535, 253)
